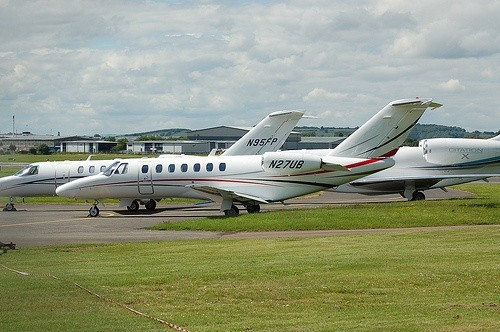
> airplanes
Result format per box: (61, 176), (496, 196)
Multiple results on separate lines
(0, 109), (320, 213)
(345, 132), (500, 202)
(55, 97), (442, 217)
